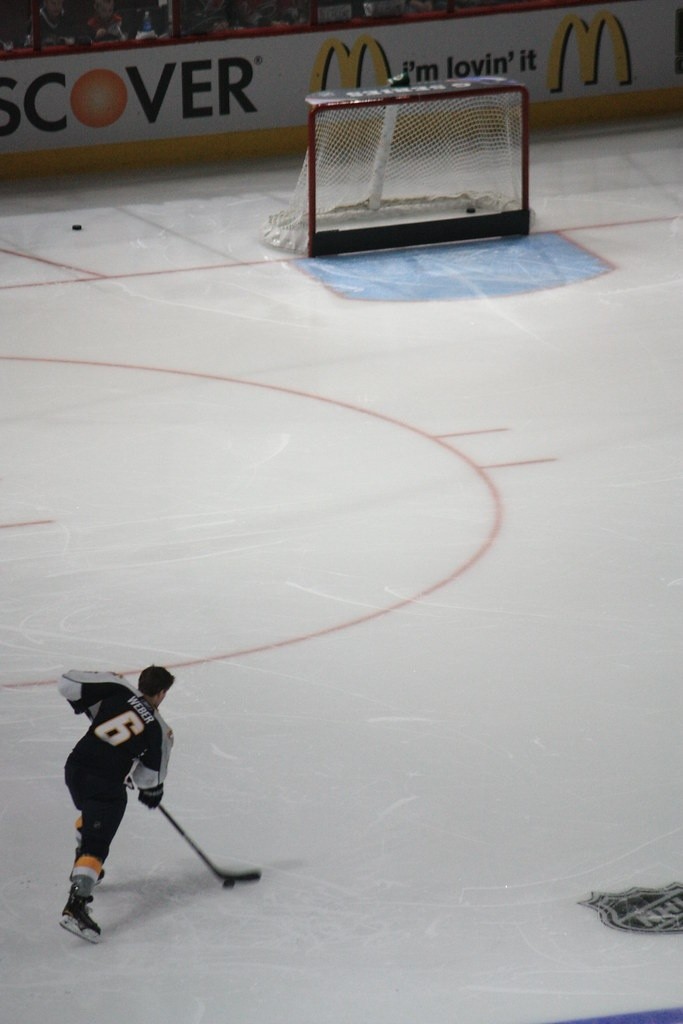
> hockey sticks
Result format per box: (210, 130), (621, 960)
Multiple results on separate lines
(132, 776), (261, 880)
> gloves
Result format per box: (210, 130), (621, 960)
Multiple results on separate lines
(137, 782), (164, 809)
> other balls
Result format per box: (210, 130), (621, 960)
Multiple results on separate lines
(71, 224), (81, 231)
(466, 207), (475, 213)
(221, 879), (236, 889)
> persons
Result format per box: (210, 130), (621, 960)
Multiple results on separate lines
(57, 665), (176, 936)
(25, 0), (462, 50)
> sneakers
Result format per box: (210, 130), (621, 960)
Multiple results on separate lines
(58, 882), (103, 943)
(69, 847), (105, 881)
